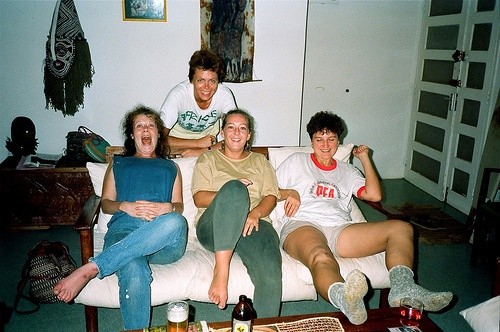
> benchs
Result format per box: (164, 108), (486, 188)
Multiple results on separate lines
(72, 145), (420, 332)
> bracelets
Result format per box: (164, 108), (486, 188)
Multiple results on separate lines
(170, 201), (176, 213)
(207, 147), (211, 151)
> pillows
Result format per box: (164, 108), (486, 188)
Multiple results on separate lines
(459, 295), (500, 332)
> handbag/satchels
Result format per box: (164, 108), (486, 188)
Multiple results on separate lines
(78, 126), (111, 163)
(13, 239), (78, 315)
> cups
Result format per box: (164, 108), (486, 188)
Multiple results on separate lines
(400, 297), (423, 327)
(167, 301), (189, 332)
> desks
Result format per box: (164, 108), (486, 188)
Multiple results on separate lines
(122, 307), (445, 332)
(0, 156), (94, 230)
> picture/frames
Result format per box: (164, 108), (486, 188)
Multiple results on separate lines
(122, 0), (167, 22)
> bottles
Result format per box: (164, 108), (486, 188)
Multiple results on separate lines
(232, 295), (252, 332)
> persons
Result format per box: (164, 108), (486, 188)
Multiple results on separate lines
(159, 50), (237, 158)
(53, 103), (188, 332)
(269, 110), (452, 325)
(191, 108), (282, 319)
